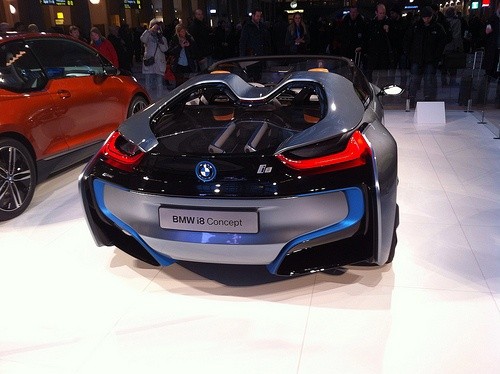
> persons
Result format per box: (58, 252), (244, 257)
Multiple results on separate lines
(0, 4), (500, 109)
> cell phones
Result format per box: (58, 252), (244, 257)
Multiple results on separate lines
(157, 26), (160, 33)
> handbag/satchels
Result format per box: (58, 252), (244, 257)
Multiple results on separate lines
(164, 67), (176, 81)
(144, 57), (155, 66)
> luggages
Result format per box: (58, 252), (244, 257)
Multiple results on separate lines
(458, 50), (486, 105)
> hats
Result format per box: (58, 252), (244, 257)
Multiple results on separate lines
(421, 6), (434, 17)
